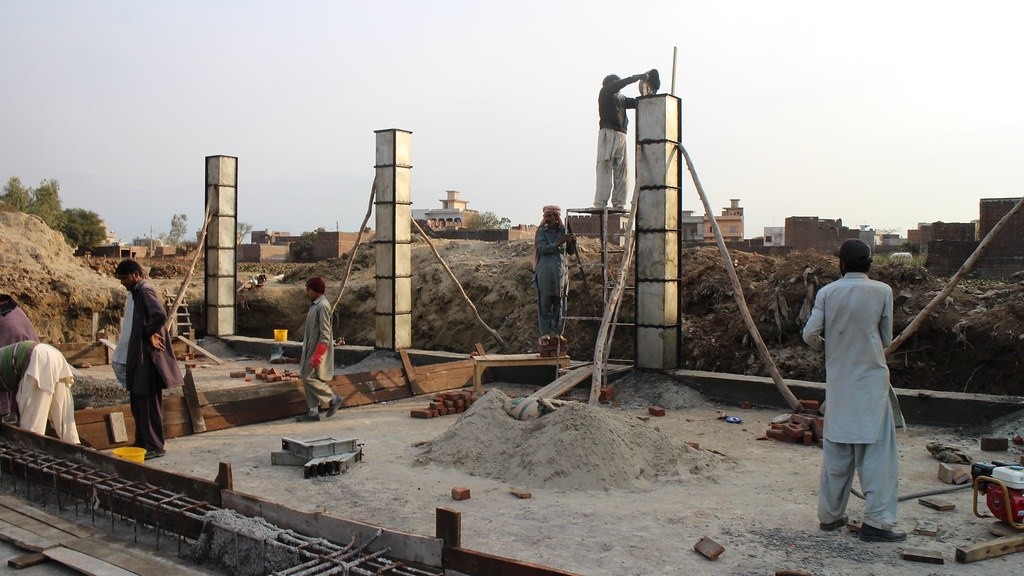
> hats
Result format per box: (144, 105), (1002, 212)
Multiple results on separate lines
(305, 276), (325, 294)
(834, 239), (871, 263)
(543, 205), (560, 217)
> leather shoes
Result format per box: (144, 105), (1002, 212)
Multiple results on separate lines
(820, 514), (848, 530)
(860, 522), (907, 542)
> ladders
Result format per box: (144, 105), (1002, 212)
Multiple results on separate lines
(166, 287), (192, 337)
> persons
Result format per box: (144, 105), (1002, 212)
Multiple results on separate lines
(531, 205), (576, 341)
(111, 259), (185, 461)
(0, 293), (42, 427)
(294, 277), (344, 423)
(593, 71), (651, 209)
(802, 237), (907, 543)
(0, 340), (82, 445)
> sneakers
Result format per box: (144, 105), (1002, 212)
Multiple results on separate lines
(295, 414), (320, 421)
(144, 450), (167, 460)
(538, 334), (567, 346)
(326, 395), (343, 417)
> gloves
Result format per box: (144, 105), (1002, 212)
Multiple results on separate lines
(309, 342), (327, 369)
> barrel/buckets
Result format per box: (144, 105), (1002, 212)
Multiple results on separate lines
(274, 329), (288, 342)
(112, 446), (146, 465)
